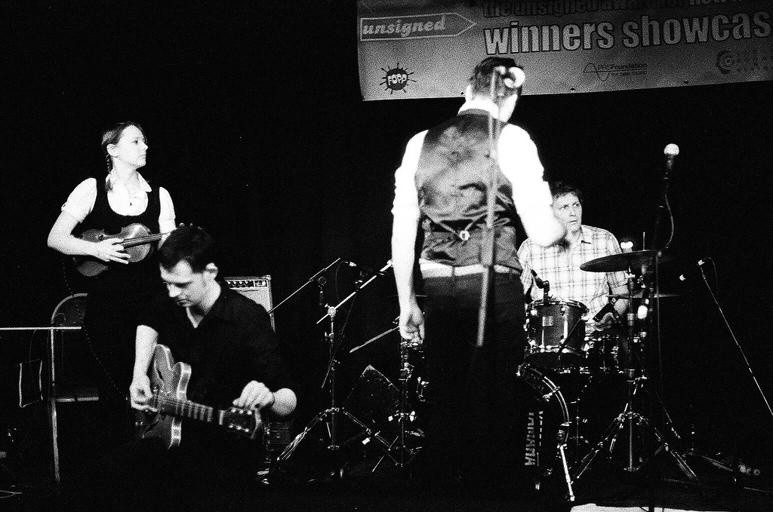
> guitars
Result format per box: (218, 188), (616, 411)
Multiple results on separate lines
(136, 344), (262, 451)
(72, 223), (191, 276)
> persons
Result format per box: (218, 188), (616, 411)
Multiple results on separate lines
(517, 183), (631, 340)
(47, 58), (179, 490)
(128, 225), (299, 512)
(390, 56), (566, 509)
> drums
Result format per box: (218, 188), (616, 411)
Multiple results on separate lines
(525, 299), (589, 369)
(411, 363), (570, 491)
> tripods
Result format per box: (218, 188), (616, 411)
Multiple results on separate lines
(569, 278), (700, 487)
(280, 304), (405, 462)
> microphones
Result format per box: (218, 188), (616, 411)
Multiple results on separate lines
(662, 143), (680, 185)
(682, 252), (717, 271)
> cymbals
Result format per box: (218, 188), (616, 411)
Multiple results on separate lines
(581, 250), (674, 271)
(608, 293), (678, 299)
